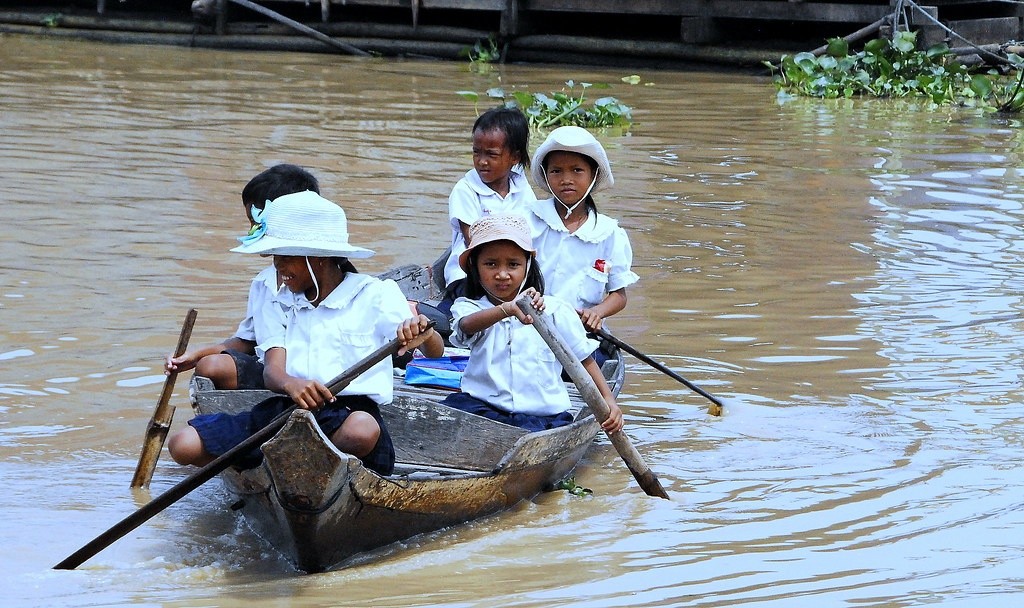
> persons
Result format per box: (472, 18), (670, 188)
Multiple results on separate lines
(167, 191), (445, 476)
(162, 165), (322, 373)
(530, 125), (640, 383)
(439, 213), (625, 435)
(446, 106), (536, 285)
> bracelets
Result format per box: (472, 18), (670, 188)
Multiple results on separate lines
(498, 304), (511, 318)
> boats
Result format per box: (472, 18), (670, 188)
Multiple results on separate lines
(188, 259), (628, 574)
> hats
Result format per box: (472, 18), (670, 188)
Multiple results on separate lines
(458, 213), (537, 303)
(530, 126), (615, 219)
(229, 190), (377, 303)
